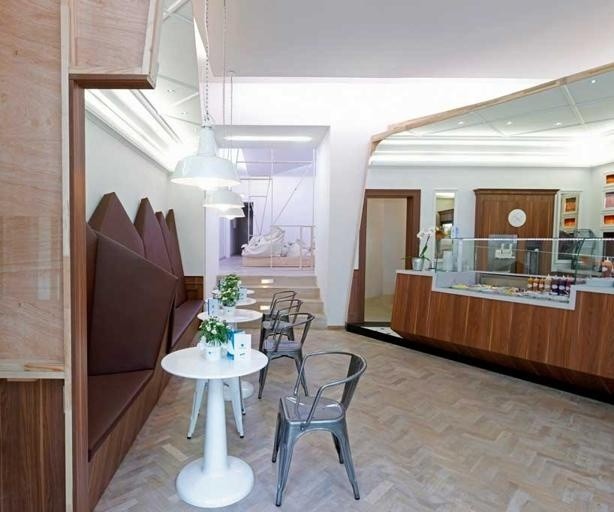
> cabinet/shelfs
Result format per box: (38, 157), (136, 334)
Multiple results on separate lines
(476, 190), (557, 283)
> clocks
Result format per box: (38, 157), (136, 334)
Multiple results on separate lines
(506, 208), (527, 227)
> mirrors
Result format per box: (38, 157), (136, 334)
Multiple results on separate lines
(434, 189), (455, 259)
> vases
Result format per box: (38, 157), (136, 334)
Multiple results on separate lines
(411, 257), (424, 270)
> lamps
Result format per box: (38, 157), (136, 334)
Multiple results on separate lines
(169, 0), (245, 222)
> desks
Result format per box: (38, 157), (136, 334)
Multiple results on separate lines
(160, 289), (269, 508)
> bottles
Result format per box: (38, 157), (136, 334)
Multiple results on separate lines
(602, 256), (612, 278)
(527, 273), (585, 298)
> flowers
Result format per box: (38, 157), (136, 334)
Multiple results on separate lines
(221, 272), (242, 288)
(398, 227), (436, 262)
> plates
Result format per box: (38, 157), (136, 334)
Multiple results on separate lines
(451, 284), (510, 294)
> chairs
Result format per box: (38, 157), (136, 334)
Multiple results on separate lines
(258, 290), (367, 505)
